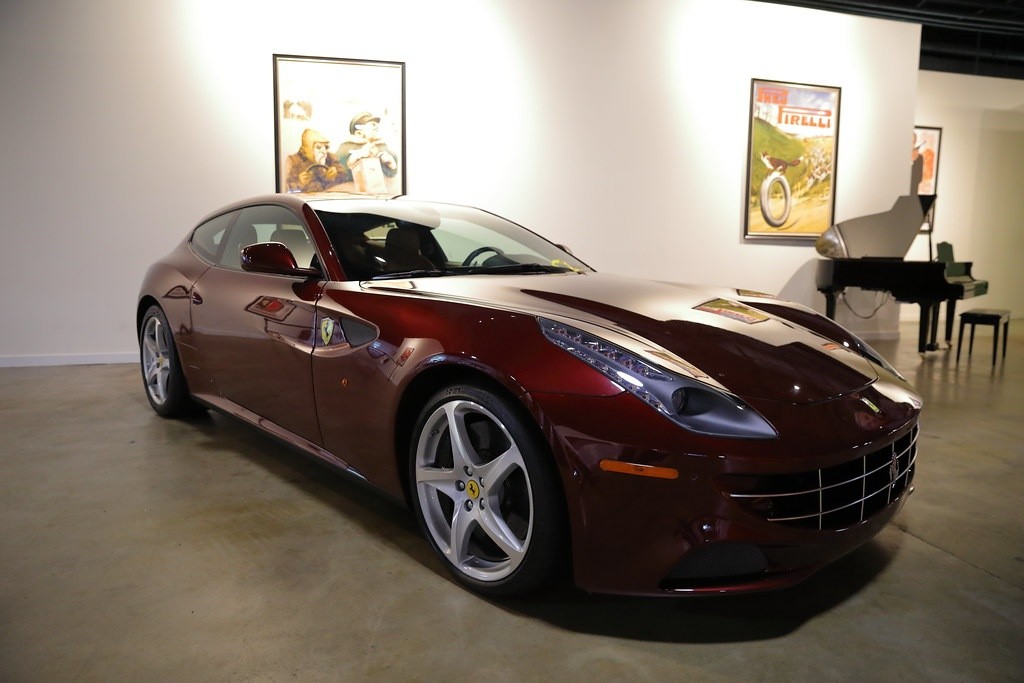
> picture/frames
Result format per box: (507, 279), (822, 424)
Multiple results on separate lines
(744, 78), (842, 241)
(912, 126), (942, 233)
(244, 296), (296, 322)
(272, 53), (407, 196)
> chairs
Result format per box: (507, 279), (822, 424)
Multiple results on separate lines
(383, 228), (435, 272)
(271, 230), (307, 250)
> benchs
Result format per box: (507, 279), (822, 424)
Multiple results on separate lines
(956, 309), (1011, 366)
(223, 220), (361, 266)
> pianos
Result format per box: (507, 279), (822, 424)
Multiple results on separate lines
(815, 193), (989, 354)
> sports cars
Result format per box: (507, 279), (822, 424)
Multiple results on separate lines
(137, 194), (923, 601)
